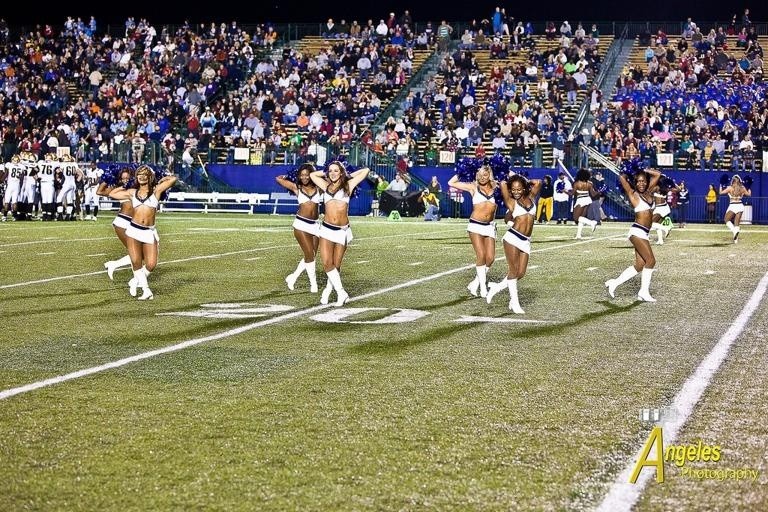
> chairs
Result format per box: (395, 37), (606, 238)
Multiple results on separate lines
(1, 19), (767, 172)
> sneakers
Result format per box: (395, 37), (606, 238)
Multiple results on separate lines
(2, 212), (97, 221)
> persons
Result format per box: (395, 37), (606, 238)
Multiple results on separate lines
(276, 164), (323, 293)
(447, 164), (504, 298)
(310, 161), (371, 306)
(110, 165), (176, 301)
(96, 164), (145, 280)
(2, 2), (768, 242)
(485, 173), (542, 314)
(605, 168), (661, 302)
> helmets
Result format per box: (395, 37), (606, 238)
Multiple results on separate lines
(11, 152), (74, 161)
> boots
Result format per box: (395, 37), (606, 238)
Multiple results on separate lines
(605, 266), (638, 298)
(726, 221), (739, 240)
(104, 255), (153, 300)
(651, 222), (671, 238)
(467, 267), (523, 315)
(285, 258), (349, 306)
(734, 226), (739, 243)
(637, 268), (656, 301)
(655, 228), (664, 245)
(574, 216), (598, 239)
